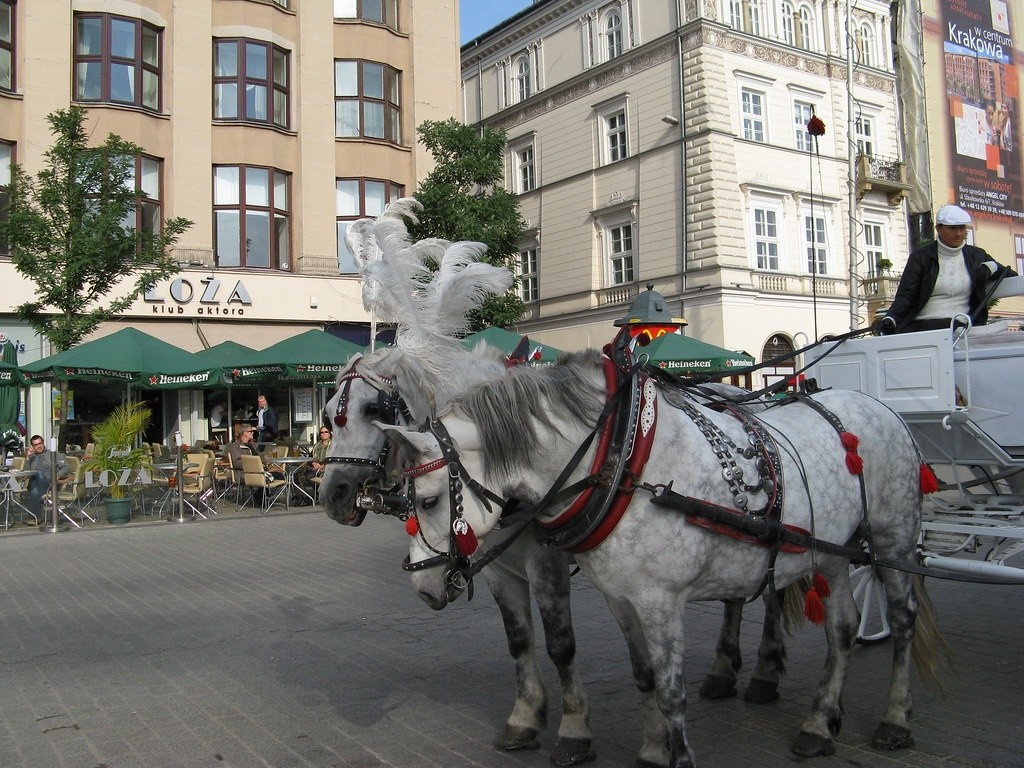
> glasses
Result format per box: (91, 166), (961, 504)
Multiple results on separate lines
(320, 429), (329, 435)
(245, 429), (253, 434)
(32, 442), (44, 447)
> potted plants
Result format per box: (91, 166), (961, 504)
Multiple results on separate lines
(76, 398), (158, 524)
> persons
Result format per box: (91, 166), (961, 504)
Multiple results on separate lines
(877, 205), (1018, 336)
(211, 401), (233, 440)
(255, 394), (278, 451)
(231, 423), (284, 508)
(26, 434), (69, 527)
(290, 426), (332, 507)
(982, 99), (998, 145)
(243, 400), (259, 418)
(998, 102), (1013, 152)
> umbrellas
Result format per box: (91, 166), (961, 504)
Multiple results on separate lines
(633, 332), (755, 390)
(459, 325), (571, 370)
(0, 341), (21, 466)
(18, 326), (234, 451)
(193, 341), (312, 445)
(228, 329), (372, 447)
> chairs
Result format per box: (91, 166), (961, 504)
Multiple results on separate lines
(0, 427), (323, 530)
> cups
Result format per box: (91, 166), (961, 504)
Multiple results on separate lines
(293, 446), (300, 460)
(271, 446), (278, 459)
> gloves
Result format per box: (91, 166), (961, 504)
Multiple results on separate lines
(977, 264), (991, 280)
(879, 319), (894, 332)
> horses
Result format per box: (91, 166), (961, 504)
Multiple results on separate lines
(319, 341), (813, 768)
(373, 345), (962, 768)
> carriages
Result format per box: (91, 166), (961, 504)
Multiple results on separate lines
(316, 195), (1024, 767)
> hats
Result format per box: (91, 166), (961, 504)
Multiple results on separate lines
(937, 205), (971, 226)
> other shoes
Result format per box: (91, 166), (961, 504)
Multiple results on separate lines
(24, 516), (42, 526)
(290, 496), (312, 507)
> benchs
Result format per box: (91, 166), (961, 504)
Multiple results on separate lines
(950, 273), (1024, 361)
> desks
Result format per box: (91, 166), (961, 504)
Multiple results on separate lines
(263, 456), (316, 513)
(148, 462), (200, 521)
(0, 469), (40, 530)
